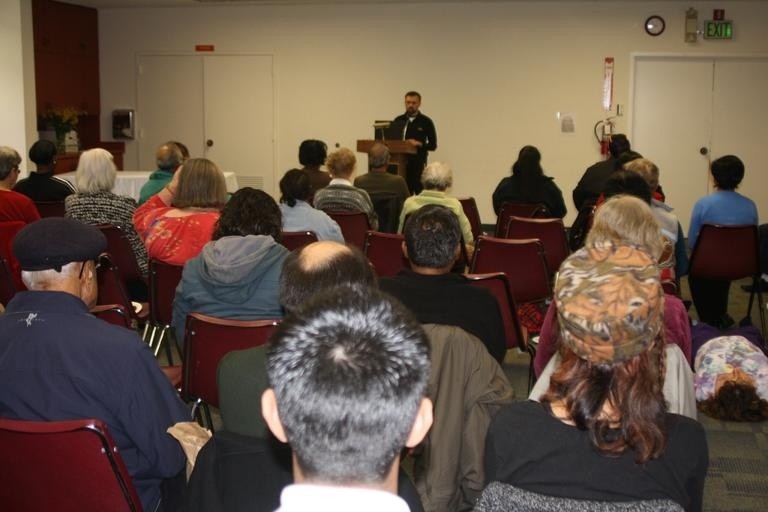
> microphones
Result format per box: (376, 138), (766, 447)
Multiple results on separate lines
(403, 120), (409, 141)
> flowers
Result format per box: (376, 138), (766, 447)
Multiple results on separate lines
(43, 104), (90, 132)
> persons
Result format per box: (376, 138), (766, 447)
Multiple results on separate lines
(0, 91), (768, 512)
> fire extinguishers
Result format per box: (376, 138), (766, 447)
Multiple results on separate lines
(594, 118), (611, 155)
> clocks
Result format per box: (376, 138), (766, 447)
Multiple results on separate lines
(644, 14), (664, 36)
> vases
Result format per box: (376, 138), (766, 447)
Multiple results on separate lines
(55, 131), (81, 153)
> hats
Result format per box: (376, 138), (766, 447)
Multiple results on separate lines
(554, 241), (665, 364)
(13, 217), (108, 271)
(30, 139), (56, 163)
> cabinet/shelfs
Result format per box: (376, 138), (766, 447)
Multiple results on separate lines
(52, 140), (125, 173)
(32, 0), (100, 117)
